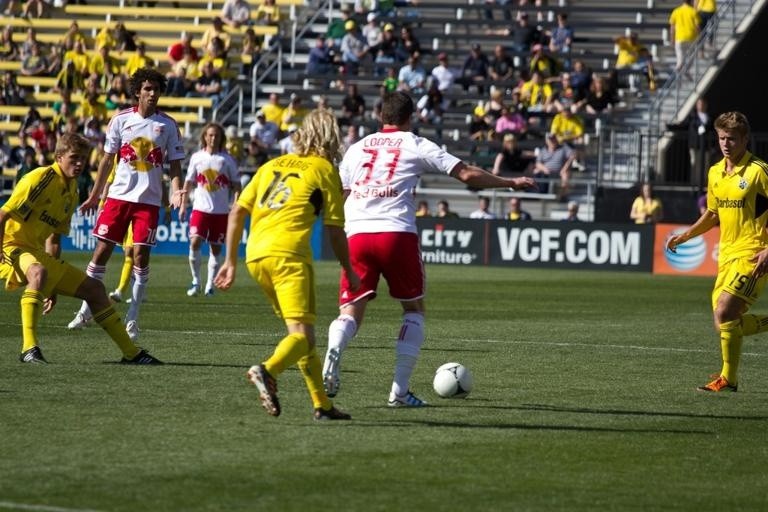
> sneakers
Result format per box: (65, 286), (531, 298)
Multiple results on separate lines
(111, 290), (142, 340)
(121, 349), (163, 364)
(315, 346), (351, 420)
(20, 346), (47, 364)
(697, 375), (738, 393)
(387, 391), (426, 407)
(68, 311), (90, 329)
(248, 364), (281, 416)
(187, 282), (215, 296)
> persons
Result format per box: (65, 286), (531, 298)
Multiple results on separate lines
(69, 69), (189, 338)
(180, 125), (243, 297)
(323, 92), (540, 406)
(666, 113), (768, 394)
(0, 133), (169, 366)
(108, 220), (138, 304)
(214, 111), (360, 422)
(1, 0), (718, 226)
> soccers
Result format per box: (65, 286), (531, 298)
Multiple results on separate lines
(432, 360), (473, 400)
